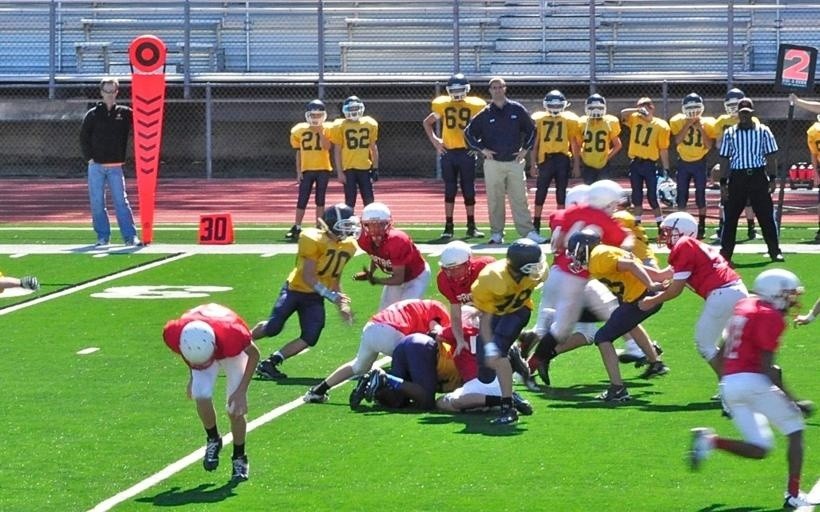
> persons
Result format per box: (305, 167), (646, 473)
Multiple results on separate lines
(788, 93), (820, 114)
(719, 97), (784, 267)
(250, 202), (359, 380)
(0, 272), (39, 293)
(518, 179), (749, 402)
(285, 98), (334, 238)
(423, 73), (488, 238)
(669, 92), (718, 239)
(578, 93), (624, 186)
(301, 240), (549, 424)
(163, 302), (260, 480)
(79, 78), (142, 246)
(530, 89), (586, 235)
(330, 95), (379, 208)
(806, 115), (820, 243)
(463, 78), (551, 245)
(688, 268), (820, 507)
(352, 203), (431, 313)
(709, 88), (763, 240)
(621, 96), (671, 235)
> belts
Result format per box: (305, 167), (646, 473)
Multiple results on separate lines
(731, 167), (763, 175)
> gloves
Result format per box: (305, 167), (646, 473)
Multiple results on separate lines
(369, 169), (378, 180)
(663, 169), (670, 179)
(639, 106), (649, 116)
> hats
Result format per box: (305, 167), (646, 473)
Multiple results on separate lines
(738, 97), (755, 113)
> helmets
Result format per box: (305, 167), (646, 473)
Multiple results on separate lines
(564, 229), (600, 274)
(584, 93), (606, 119)
(682, 92), (704, 119)
(565, 179), (623, 215)
(724, 88), (744, 116)
(305, 99), (327, 126)
(543, 90), (568, 116)
(446, 73), (471, 102)
(179, 320), (216, 370)
(659, 180), (678, 207)
(506, 238), (546, 274)
(437, 240), (472, 277)
(655, 212), (698, 248)
(317, 203), (393, 248)
(343, 95), (365, 120)
(753, 268), (805, 311)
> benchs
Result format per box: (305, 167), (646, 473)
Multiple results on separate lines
(0, 0), (820, 79)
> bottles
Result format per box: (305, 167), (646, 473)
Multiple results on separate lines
(789, 164), (816, 181)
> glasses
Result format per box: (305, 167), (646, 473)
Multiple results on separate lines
(102, 88), (116, 94)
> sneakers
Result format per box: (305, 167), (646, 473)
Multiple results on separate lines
(443, 225), (454, 237)
(231, 455), (249, 482)
(507, 332), (549, 391)
(467, 224), (485, 238)
(594, 384), (630, 401)
(783, 491), (811, 509)
(490, 392), (532, 426)
(658, 229), (667, 239)
(690, 427), (719, 465)
(750, 233), (764, 240)
(95, 238), (109, 246)
(125, 235), (142, 246)
(286, 224), (301, 238)
(303, 386), (329, 403)
(697, 224), (720, 240)
(21, 275), (40, 289)
(618, 340), (669, 379)
(488, 231), (502, 244)
(525, 228), (547, 244)
(204, 435), (222, 471)
(351, 368), (389, 410)
(771, 252), (784, 261)
(256, 360), (287, 381)
